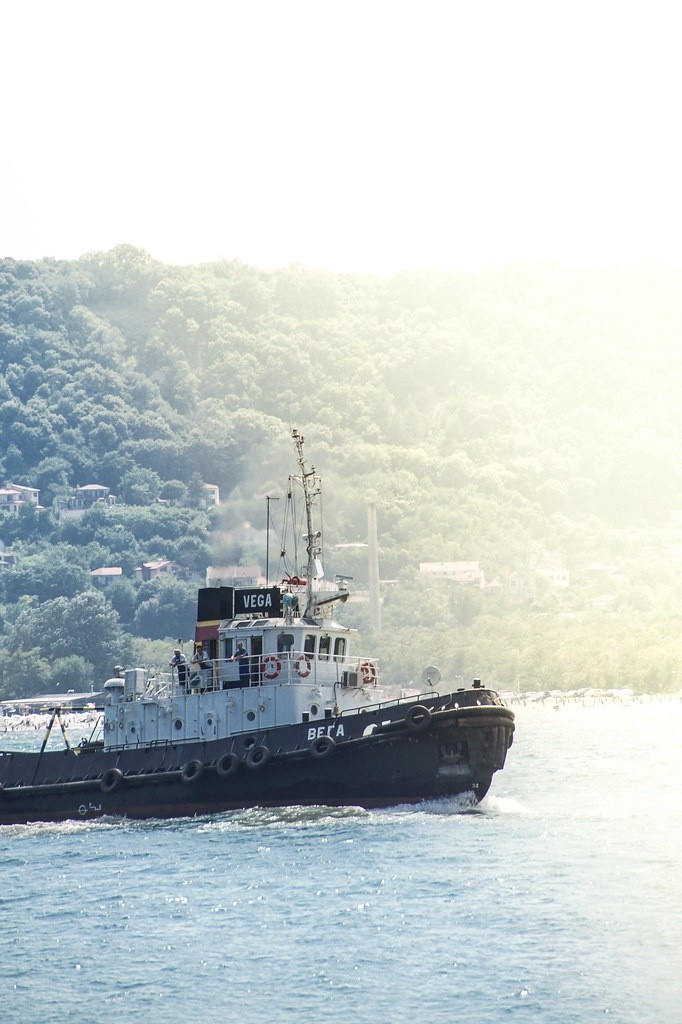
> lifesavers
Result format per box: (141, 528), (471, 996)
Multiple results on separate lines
(216, 752), (240, 777)
(295, 656), (312, 677)
(359, 662), (376, 683)
(99, 768), (124, 794)
(181, 760), (206, 785)
(404, 705), (432, 731)
(309, 735), (336, 762)
(245, 745), (272, 771)
(262, 656), (282, 679)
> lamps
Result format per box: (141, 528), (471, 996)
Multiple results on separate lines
(283, 593), (299, 608)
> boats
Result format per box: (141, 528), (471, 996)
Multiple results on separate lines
(0, 429), (515, 826)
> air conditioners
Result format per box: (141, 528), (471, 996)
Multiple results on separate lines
(343, 670), (363, 689)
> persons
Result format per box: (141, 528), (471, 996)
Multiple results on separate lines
(168, 649), (191, 694)
(190, 645), (213, 692)
(231, 642), (249, 687)
